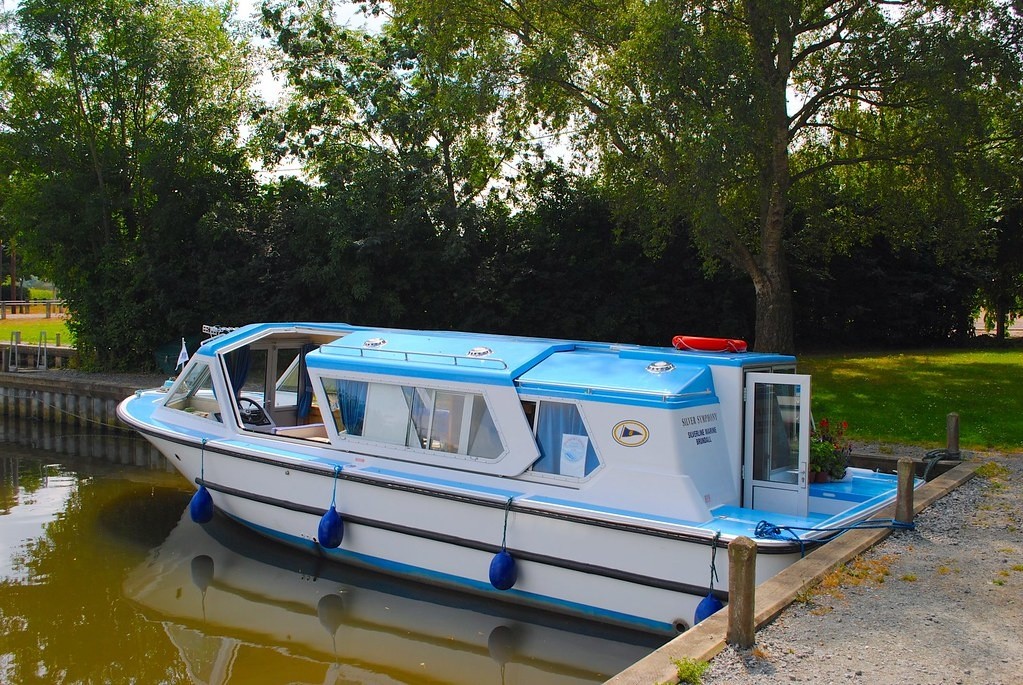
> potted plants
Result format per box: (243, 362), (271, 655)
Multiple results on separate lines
(789, 437), (835, 483)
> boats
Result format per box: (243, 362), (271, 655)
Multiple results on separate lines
(115, 320), (928, 642)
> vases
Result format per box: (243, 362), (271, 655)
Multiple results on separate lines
(817, 472), (829, 482)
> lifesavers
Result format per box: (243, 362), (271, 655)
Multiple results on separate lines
(671, 336), (749, 354)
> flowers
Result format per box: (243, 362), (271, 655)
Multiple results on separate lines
(810, 418), (852, 478)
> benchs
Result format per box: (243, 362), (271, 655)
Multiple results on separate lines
(272, 423), (328, 438)
